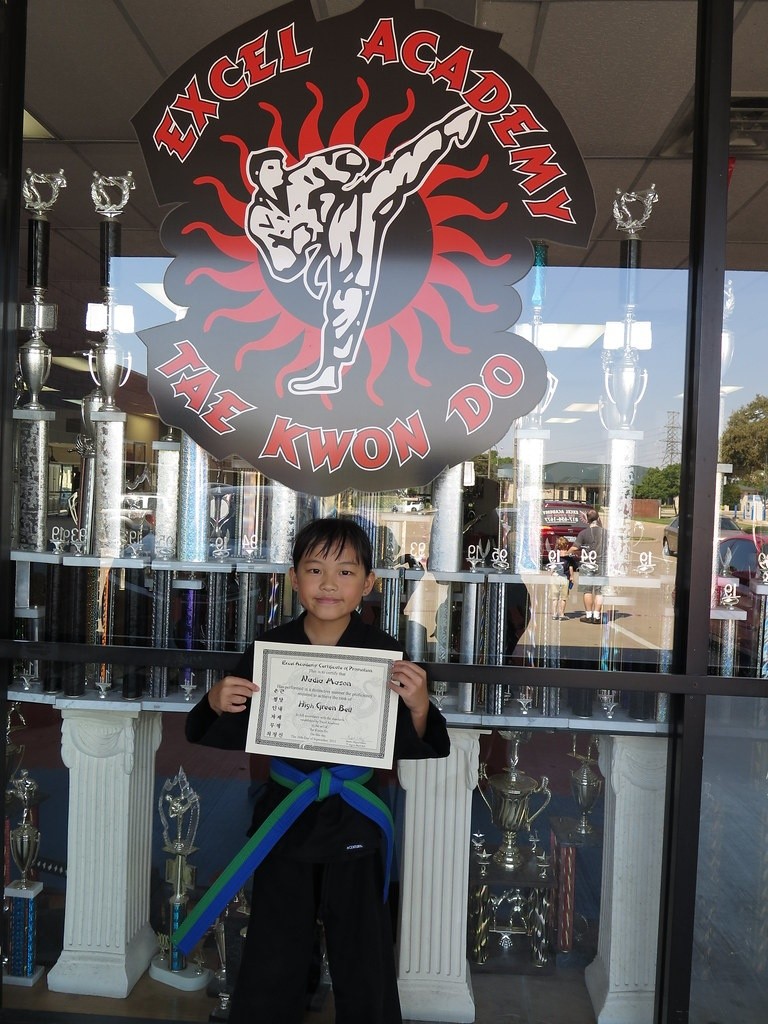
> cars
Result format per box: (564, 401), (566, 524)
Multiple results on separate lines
(391, 499), (425, 514)
(673, 535), (768, 665)
(108, 484), (337, 647)
(661, 515), (748, 555)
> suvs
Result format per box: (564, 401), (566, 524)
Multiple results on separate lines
(538, 498), (596, 570)
(120, 494), (167, 535)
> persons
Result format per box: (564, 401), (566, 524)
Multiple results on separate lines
(550, 510), (608, 624)
(183, 519), (450, 1024)
(424, 476), (531, 654)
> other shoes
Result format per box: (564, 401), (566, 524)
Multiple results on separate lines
(593, 617), (602, 623)
(580, 615), (593, 623)
(552, 615), (559, 620)
(559, 615), (569, 621)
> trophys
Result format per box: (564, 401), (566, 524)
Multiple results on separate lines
(721, 276), (736, 385)
(0, 701), (43, 987)
(515, 239), (558, 427)
(15, 167), (136, 557)
(472, 736), (602, 966)
(156, 767), (249, 1024)
(597, 182), (660, 430)
